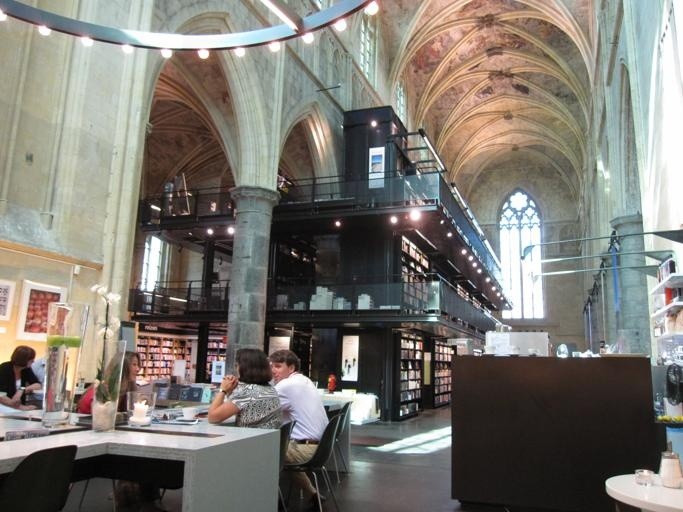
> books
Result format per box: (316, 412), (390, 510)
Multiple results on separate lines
(454, 289), (491, 326)
(399, 333), (423, 415)
(408, 190), (426, 206)
(275, 286), (400, 312)
(401, 237), (429, 315)
(434, 340), (454, 405)
(135, 335), (225, 383)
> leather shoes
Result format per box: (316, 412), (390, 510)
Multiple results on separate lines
(306, 492), (325, 510)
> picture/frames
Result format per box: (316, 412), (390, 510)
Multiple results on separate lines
(16, 279), (67, 344)
(0, 279), (16, 322)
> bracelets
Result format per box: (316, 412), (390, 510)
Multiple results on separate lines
(218, 389), (226, 394)
(17, 385), (27, 393)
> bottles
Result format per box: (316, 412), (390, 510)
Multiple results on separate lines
(658, 440), (682, 489)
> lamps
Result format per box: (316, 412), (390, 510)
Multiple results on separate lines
(398, 128), (502, 272)
(0, 0), (378, 61)
(522, 228), (683, 283)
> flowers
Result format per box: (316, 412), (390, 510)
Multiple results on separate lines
(91, 282), (126, 400)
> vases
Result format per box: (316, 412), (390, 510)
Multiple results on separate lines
(90, 332), (127, 434)
(40, 301), (90, 428)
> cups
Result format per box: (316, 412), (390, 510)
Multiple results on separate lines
(126, 391), (157, 428)
(182, 407), (198, 420)
(635, 469), (654, 485)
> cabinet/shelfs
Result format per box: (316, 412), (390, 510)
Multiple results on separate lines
(342, 106), (408, 204)
(386, 234), (498, 422)
(132, 334), (229, 389)
(648, 273), (682, 341)
(309, 285), (375, 310)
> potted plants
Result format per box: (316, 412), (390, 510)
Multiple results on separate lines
(330, 367), (344, 391)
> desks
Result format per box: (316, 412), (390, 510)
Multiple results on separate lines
(319, 389), (379, 424)
(606, 472), (683, 511)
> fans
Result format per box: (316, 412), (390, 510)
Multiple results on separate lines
(664, 364), (683, 405)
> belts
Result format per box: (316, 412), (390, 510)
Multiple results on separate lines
(295, 438), (319, 445)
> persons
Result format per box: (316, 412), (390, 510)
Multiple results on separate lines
(207, 346), (282, 430)
(269, 349), (331, 511)
(76, 350), (141, 414)
(0, 344), (42, 411)
